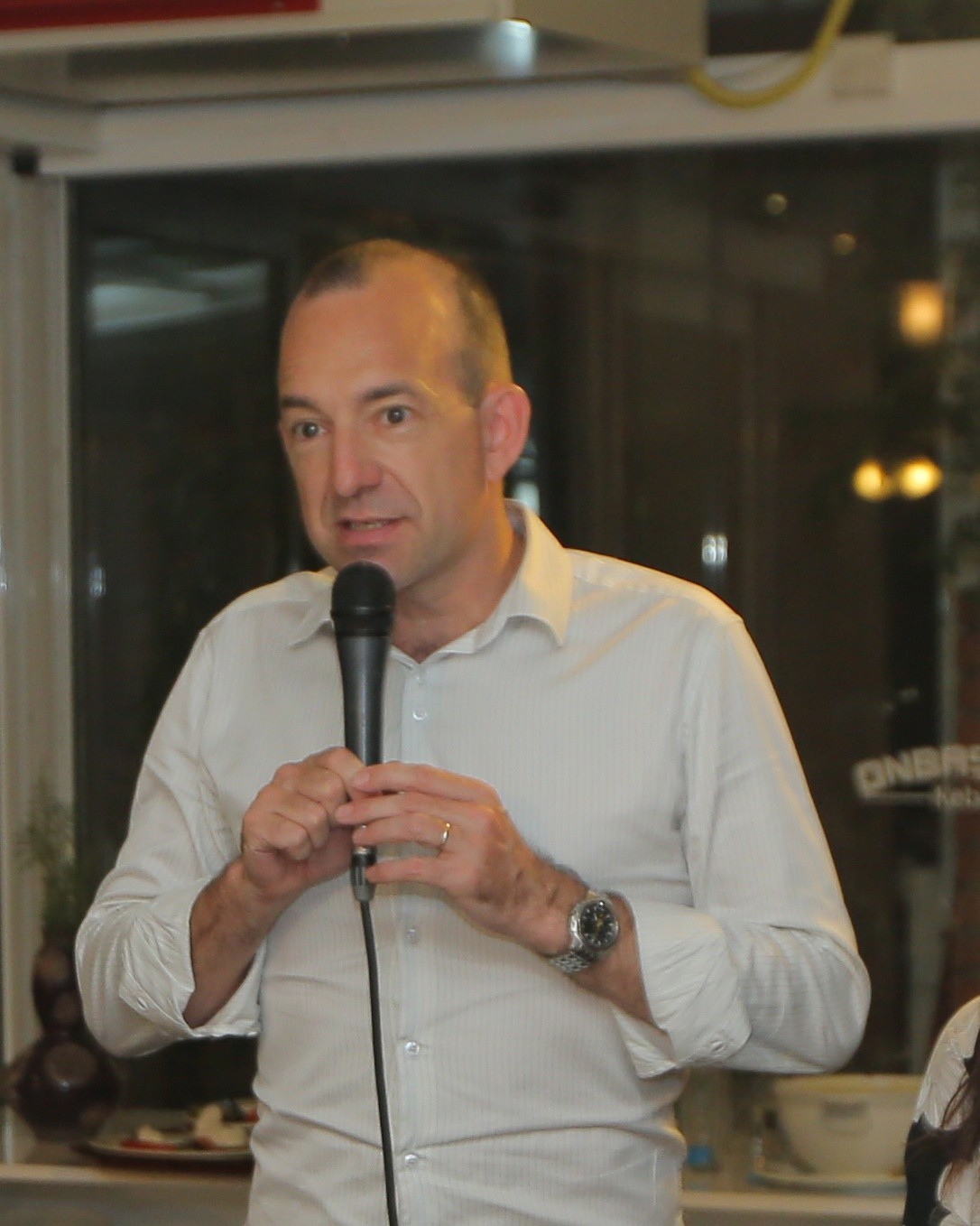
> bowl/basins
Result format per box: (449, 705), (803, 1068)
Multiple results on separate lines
(773, 1074), (922, 1178)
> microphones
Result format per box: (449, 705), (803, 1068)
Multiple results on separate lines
(331, 559), (394, 900)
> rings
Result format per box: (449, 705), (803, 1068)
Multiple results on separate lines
(436, 822), (451, 851)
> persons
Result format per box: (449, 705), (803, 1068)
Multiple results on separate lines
(903, 997), (980, 1226)
(74, 234), (872, 1226)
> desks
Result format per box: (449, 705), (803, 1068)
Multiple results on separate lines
(0, 1156), (913, 1226)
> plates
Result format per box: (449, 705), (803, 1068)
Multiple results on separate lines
(753, 1166), (907, 1191)
(87, 1098), (263, 1163)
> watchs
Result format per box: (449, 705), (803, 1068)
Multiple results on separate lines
(538, 888), (620, 975)
